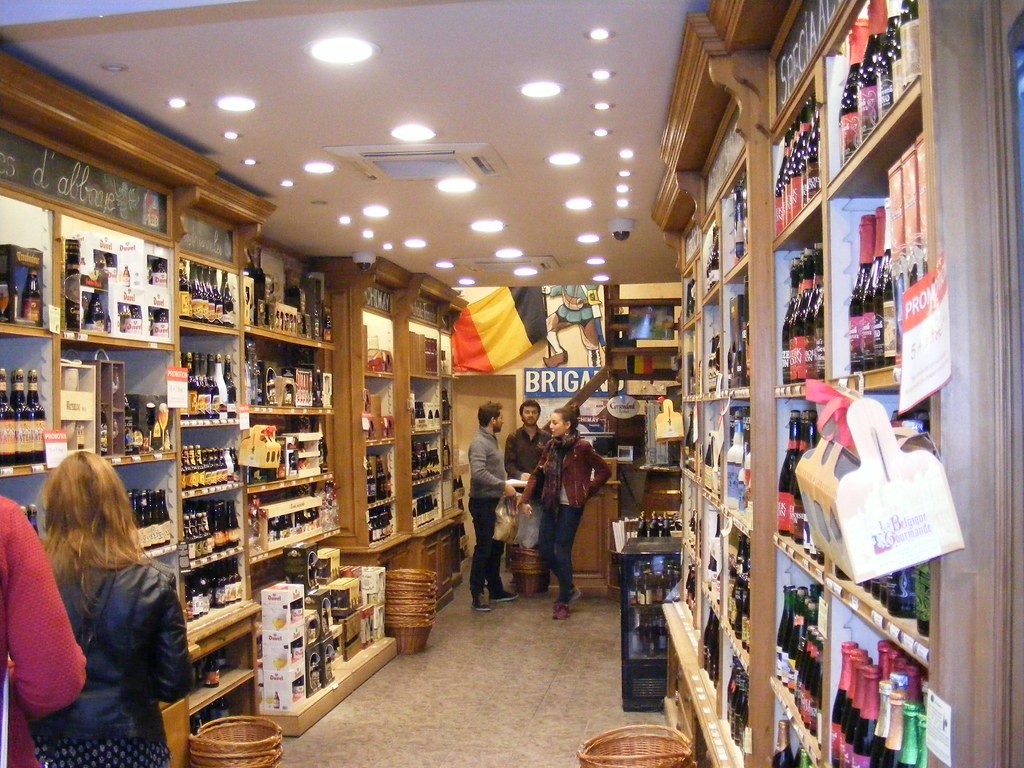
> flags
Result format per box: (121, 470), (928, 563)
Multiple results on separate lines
(452, 286), (546, 373)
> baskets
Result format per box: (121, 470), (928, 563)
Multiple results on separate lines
(509, 546), (550, 594)
(189, 715), (284, 768)
(577, 724), (698, 768)
(385, 568), (438, 654)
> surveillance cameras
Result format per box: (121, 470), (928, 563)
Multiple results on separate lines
(608, 218), (634, 242)
(351, 251), (376, 271)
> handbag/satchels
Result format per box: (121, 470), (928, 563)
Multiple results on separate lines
(492, 492), (521, 545)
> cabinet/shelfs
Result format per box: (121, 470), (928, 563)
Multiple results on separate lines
(439, 294), (475, 561)
(169, 176), (276, 730)
(649, 0), (1024, 768)
(411, 274), (460, 609)
(306, 255), (411, 577)
(0, 46), (193, 768)
(247, 241), (339, 558)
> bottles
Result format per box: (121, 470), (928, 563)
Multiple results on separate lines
(0, 251), (464, 740)
(631, 0), (931, 768)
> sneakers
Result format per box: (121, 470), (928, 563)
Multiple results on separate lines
(473, 594), (491, 612)
(489, 590), (519, 602)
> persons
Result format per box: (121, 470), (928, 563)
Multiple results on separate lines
(27, 450), (192, 768)
(520, 404), (613, 620)
(504, 400), (553, 588)
(467, 402), (516, 611)
(0, 495), (87, 768)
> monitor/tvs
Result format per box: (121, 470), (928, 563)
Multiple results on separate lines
(579, 432), (617, 458)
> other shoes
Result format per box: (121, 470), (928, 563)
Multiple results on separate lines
(552, 587), (582, 620)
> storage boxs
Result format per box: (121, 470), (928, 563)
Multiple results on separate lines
(788, 385), (967, 584)
(658, 397), (683, 440)
(259, 562), (387, 705)
